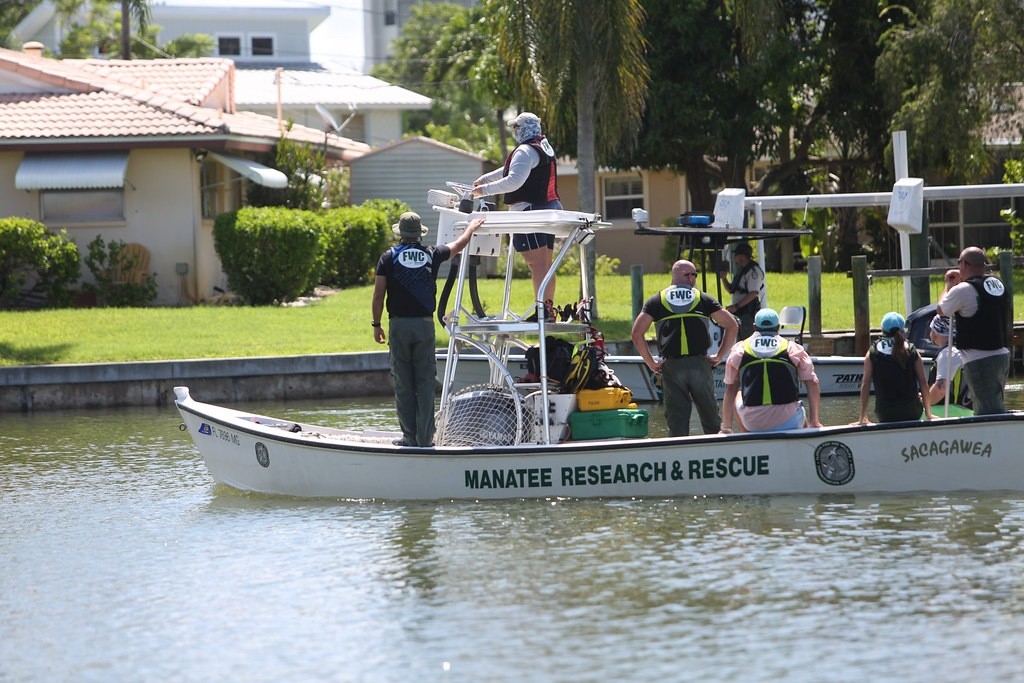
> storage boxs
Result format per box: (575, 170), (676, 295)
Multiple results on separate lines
(568, 408), (648, 440)
(577, 387), (634, 409)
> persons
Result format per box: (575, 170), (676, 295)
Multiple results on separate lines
(918, 314), (973, 411)
(631, 259), (739, 438)
(472, 112), (563, 323)
(718, 308), (823, 434)
(720, 243), (768, 342)
(937, 246), (1010, 414)
(849, 312), (938, 424)
(372, 212), (485, 447)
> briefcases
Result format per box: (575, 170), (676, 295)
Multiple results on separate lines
(577, 387), (632, 411)
(569, 408), (649, 440)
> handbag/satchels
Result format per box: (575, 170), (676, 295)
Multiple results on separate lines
(515, 335), (623, 392)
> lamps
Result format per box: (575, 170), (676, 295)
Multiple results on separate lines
(576, 229), (596, 245)
(195, 148), (208, 162)
(631, 208), (648, 225)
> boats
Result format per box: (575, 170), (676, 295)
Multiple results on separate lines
(171, 178), (1024, 503)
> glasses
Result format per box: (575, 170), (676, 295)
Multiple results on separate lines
(958, 258), (971, 265)
(683, 272), (698, 278)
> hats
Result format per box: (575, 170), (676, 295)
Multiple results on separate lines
(507, 120), (518, 127)
(393, 212), (428, 239)
(930, 314), (957, 337)
(732, 243), (754, 259)
(882, 311), (906, 333)
(754, 308), (779, 329)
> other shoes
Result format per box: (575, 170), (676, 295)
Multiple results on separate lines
(392, 438), (406, 446)
(524, 298), (555, 323)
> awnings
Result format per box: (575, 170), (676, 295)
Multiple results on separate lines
(196, 148), (289, 189)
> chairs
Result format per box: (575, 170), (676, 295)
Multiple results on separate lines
(778, 305), (807, 344)
(100, 243), (149, 293)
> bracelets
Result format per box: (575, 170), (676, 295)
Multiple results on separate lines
(371, 321), (380, 327)
(720, 423), (732, 432)
(736, 304), (740, 309)
